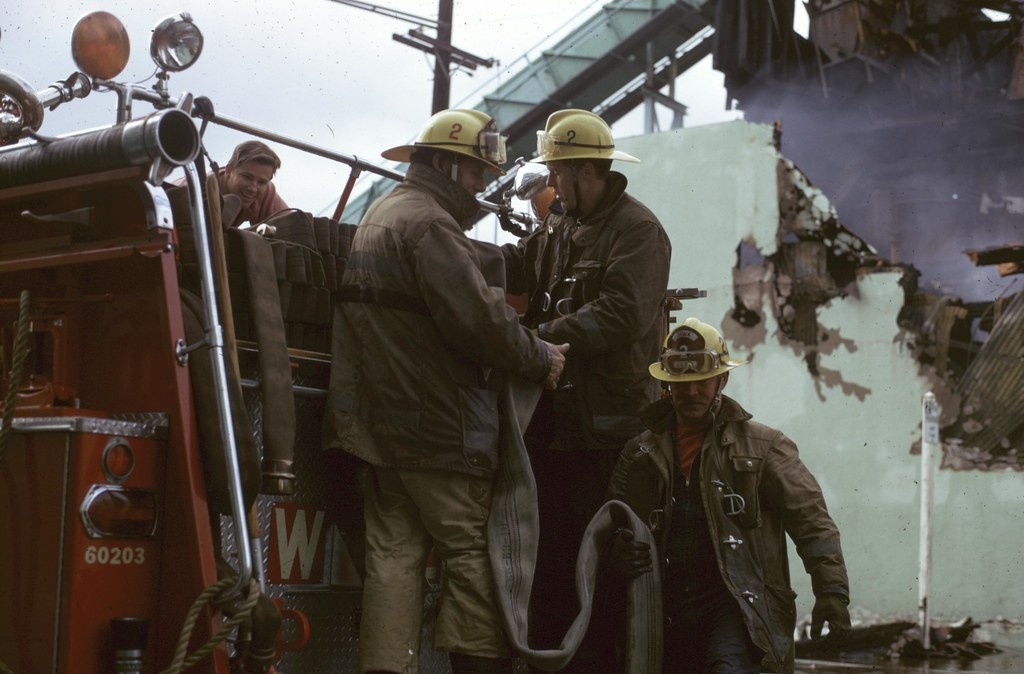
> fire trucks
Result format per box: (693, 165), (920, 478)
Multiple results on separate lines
(1, 0), (714, 674)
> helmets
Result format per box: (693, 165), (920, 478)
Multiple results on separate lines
(530, 109), (640, 163)
(649, 321), (748, 382)
(380, 109), (506, 177)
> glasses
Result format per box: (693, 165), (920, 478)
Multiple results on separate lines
(413, 131), (505, 166)
(658, 349), (729, 374)
(535, 130), (616, 159)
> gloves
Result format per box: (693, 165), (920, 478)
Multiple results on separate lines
(609, 529), (653, 578)
(810, 595), (851, 656)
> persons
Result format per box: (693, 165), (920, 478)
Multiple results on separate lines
(206, 141), (294, 228)
(603, 317), (853, 674)
(320, 109), (673, 674)
